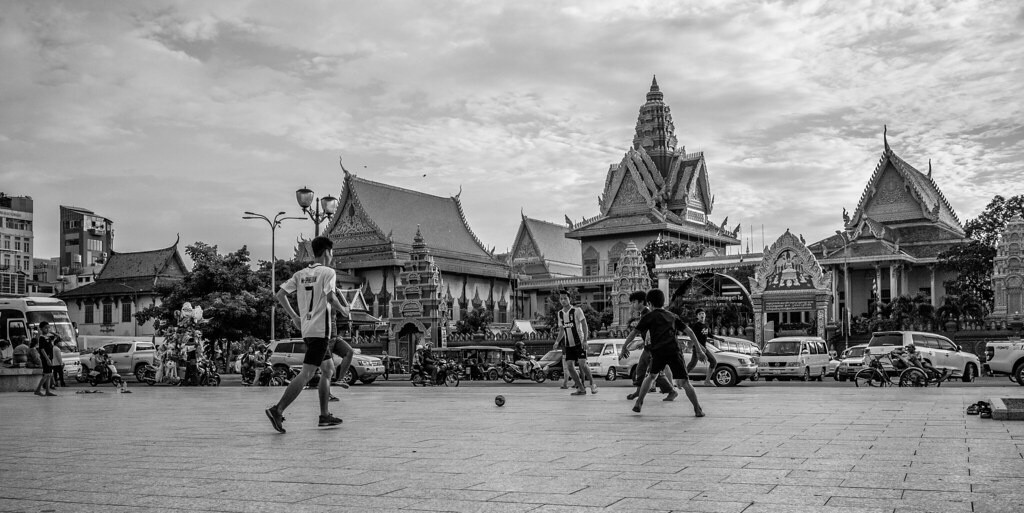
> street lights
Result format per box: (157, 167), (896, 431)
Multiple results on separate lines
(519, 269), (526, 320)
(242, 212), (307, 340)
(296, 186), (337, 237)
(120, 283), (137, 336)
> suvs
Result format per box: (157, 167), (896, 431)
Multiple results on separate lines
(826, 331), (981, 382)
(615, 336), (757, 387)
(267, 338), (385, 385)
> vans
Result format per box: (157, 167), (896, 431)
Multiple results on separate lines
(706, 336), (830, 382)
(538, 350), (562, 381)
(586, 337), (630, 381)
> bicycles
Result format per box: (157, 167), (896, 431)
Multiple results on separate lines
(854, 354), (929, 387)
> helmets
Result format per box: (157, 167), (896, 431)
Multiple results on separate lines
(515, 341), (524, 350)
(98, 347), (105, 353)
(93, 349), (98, 354)
(416, 345), (423, 350)
(425, 343), (431, 350)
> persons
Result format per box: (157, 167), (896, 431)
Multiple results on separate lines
(263, 236), (353, 434)
(0, 321), (69, 396)
(240, 346), (273, 387)
(865, 344), (942, 386)
(412, 342), (437, 385)
(512, 341), (531, 373)
(618, 290), (725, 417)
(552, 290), (598, 395)
(94, 347), (109, 379)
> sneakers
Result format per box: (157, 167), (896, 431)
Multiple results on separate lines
(318, 414), (343, 425)
(329, 394), (339, 401)
(335, 379), (349, 389)
(266, 405), (286, 433)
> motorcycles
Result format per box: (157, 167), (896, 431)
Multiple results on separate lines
(241, 357), (283, 386)
(410, 354), (459, 387)
(501, 352), (547, 383)
(89, 359), (121, 386)
(144, 349), (221, 386)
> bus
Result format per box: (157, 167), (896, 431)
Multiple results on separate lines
(432, 346), (514, 379)
(0, 297), (83, 380)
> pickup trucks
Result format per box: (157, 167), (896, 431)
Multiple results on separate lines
(983, 339), (1024, 385)
(76, 342), (156, 383)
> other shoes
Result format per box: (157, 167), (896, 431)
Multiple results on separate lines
(967, 401), (991, 417)
(121, 390), (132, 393)
(561, 383), (598, 395)
(76, 389), (104, 393)
(650, 388), (656, 392)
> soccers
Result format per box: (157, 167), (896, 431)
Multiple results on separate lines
(494, 395), (506, 406)
